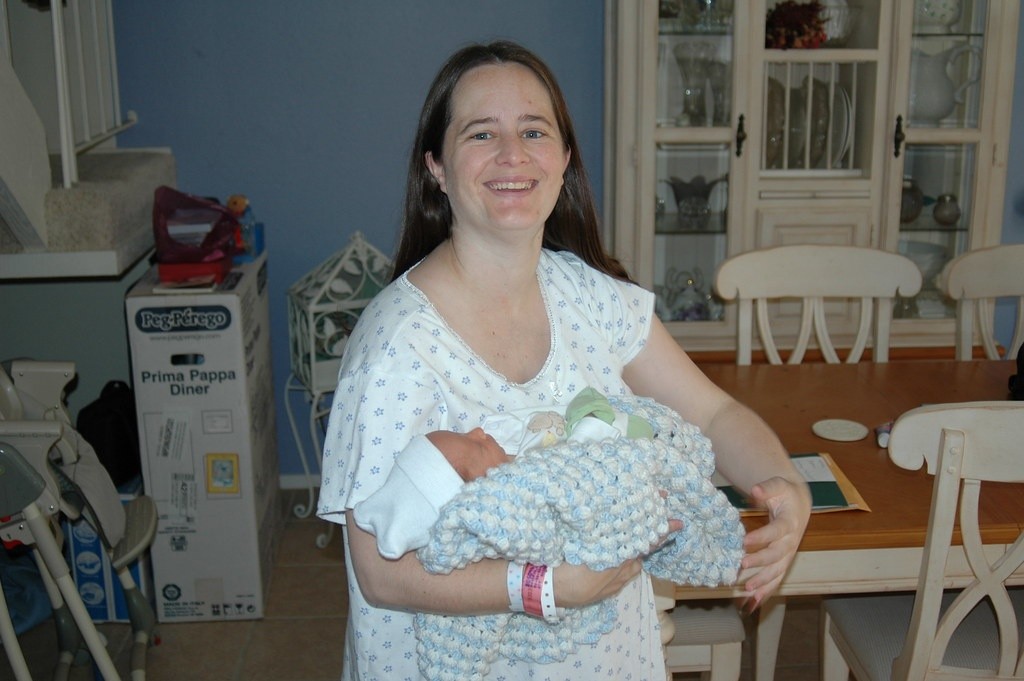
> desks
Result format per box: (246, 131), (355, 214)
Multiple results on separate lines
(678, 362), (1024, 681)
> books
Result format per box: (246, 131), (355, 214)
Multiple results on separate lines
(712, 451), (872, 516)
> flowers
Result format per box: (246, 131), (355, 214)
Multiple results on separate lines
(764, 1), (838, 51)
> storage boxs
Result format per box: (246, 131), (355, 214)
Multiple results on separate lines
(124, 256), (286, 623)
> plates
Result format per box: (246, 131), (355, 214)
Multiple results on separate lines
(812, 419), (870, 441)
(765, 77), (852, 169)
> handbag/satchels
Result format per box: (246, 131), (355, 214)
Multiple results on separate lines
(75, 380), (141, 491)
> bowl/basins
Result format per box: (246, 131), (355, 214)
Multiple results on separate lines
(820, 6), (863, 47)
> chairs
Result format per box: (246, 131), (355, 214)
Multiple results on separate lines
(0, 360), (159, 681)
(713, 245), (923, 365)
(934, 242), (1024, 360)
(820, 401), (1024, 681)
(665, 598), (746, 681)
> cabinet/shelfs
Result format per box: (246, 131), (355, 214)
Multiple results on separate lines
(602, 0), (1020, 349)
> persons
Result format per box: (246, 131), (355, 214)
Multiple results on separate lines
(315, 40), (813, 681)
(352, 384), (746, 589)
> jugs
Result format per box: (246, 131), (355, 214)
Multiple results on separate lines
(907, 39), (984, 129)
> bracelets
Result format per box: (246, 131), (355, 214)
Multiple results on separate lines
(506, 560), (566, 624)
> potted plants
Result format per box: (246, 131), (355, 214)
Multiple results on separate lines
(660, 174), (724, 217)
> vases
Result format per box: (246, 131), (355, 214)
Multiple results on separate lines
(671, 40), (718, 128)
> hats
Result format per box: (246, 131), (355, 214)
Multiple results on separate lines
(352, 433), (469, 561)
